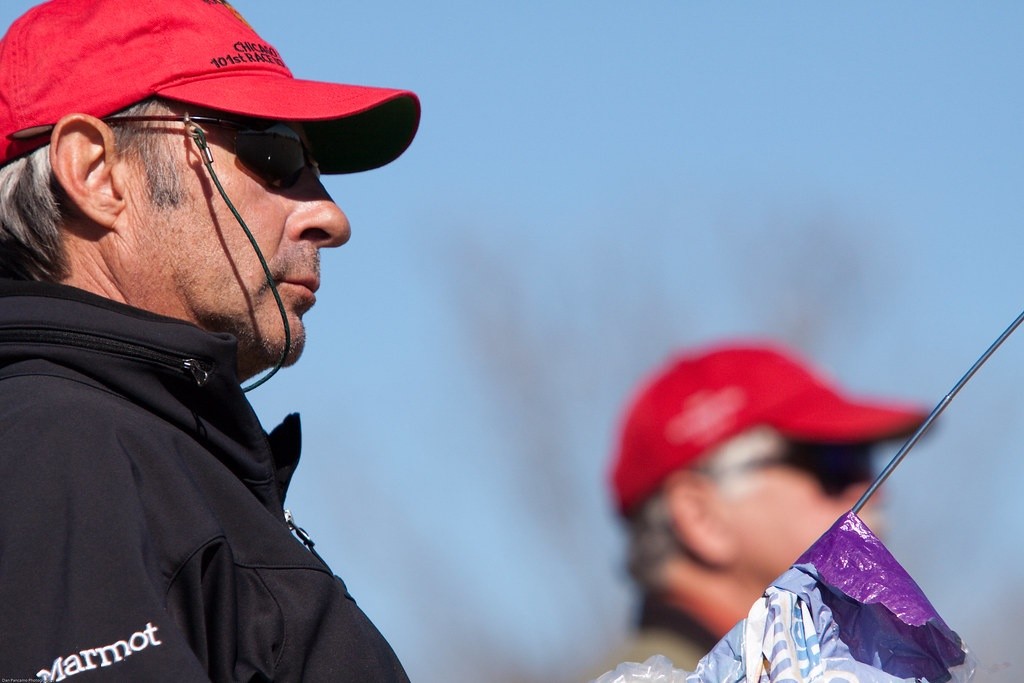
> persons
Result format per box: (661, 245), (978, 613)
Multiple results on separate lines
(582, 344), (936, 683)
(0, 0), (419, 683)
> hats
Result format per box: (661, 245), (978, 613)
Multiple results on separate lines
(0, 0), (421, 175)
(614, 348), (932, 511)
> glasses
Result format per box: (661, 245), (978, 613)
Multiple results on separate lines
(102, 116), (321, 189)
(686, 438), (876, 493)
